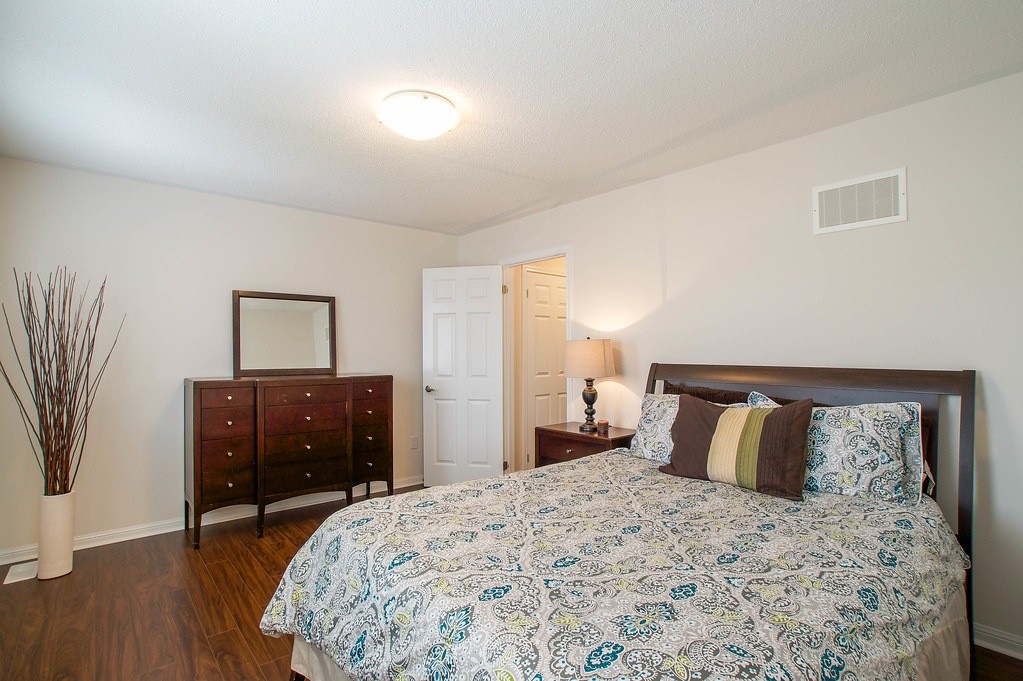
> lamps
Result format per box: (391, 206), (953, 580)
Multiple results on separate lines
(377, 91), (459, 141)
(564, 337), (616, 433)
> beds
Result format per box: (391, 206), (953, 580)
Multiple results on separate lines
(263, 448), (970, 681)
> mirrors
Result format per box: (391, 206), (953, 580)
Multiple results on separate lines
(232, 289), (337, 375)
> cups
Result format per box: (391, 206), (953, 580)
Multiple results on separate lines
(598, 420), (608, 435)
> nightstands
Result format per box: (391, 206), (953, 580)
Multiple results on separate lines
(534, 421), (637, 469)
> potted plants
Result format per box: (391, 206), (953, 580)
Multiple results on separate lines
(0, 265), (126, 580)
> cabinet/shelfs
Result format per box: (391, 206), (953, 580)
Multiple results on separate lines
(183, 373), (393, 550)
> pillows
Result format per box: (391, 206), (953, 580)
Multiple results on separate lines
(804, 406), (904, 503)
(747, 391), (923, 505)
(632, 393), (748, 464)
(658, 393), (813, 500)
